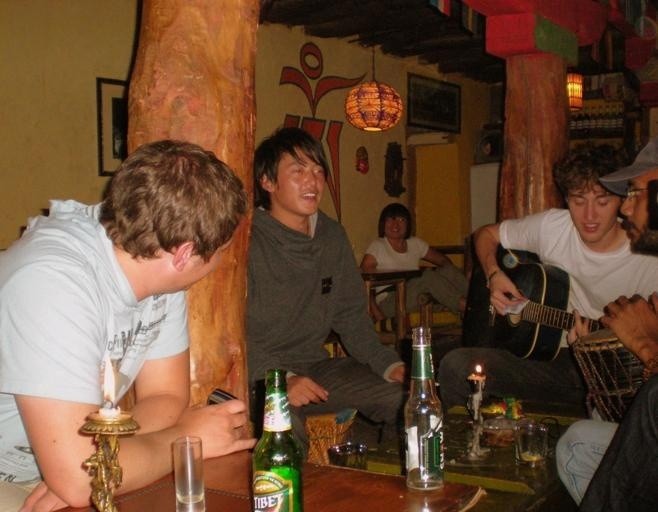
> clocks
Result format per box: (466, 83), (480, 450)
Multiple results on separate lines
(383, 140), (408, 198)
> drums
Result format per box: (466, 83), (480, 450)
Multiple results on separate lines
(571, 328), (649, 423)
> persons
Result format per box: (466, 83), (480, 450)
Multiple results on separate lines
(553, 135), (658, 512)
(0, 139), (258, 512)
(360, 201), (471, 320)
(438, 143), (658, 408)
(247, 126), (411, 459)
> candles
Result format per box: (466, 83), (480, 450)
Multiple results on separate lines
(74, 355), (141, 512)
(454, 363), (493, 464)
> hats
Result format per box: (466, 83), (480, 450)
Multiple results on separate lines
(597, 139), (658, 196)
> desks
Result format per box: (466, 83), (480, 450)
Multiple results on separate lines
(359, 267), (423, 342)
(47, 402), (590, 512)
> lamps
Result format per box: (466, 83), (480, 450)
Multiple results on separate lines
(343, 0), (404, 133)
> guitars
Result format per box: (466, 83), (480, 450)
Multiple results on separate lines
(462, 243), (607, 362)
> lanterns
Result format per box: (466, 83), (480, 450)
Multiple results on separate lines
(344, 81), (403, 133)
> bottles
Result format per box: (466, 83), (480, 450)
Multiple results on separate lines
(404, 326), (444, 491)
(569, 104), (624, 139)
(251, 368), (303, 511)
(599, 23), (625, 69)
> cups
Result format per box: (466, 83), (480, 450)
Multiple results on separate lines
(173, 436), (206, 512)
(328, 440), (369, 470)
(514, 424), (548, 469)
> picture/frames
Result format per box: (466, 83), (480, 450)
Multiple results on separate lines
(406, 71), (462, 134)
(96, 76), (130, 177)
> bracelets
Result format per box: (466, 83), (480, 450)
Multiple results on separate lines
(641, 352), (658, 382)
(481, 265), (503, 287)
